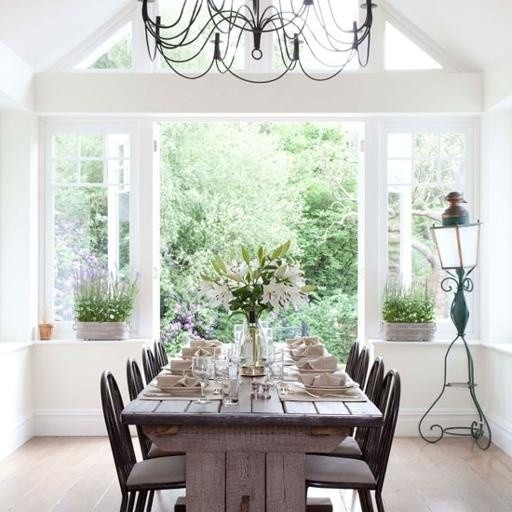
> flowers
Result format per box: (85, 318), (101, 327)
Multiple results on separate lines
(71, 267), (140, 322)
(382, 275), (440, 322)
(200, 241), (320, 365)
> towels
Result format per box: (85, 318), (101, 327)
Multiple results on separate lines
(155, 339), (224, 387)
(286, 336), (347, 388)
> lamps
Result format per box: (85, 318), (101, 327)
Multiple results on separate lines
(138, 0), (377, 84)
(418, 191), (492, 450)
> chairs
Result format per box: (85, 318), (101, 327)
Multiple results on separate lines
(329, 355), (385, 512)
(100, 372), (186, 512)
(142, 347), (160, 384)
(306, 370), (400, 512)
(345, 341), (359, 379)
(127, 357), (186, 512)
(354, 347), (369, 391)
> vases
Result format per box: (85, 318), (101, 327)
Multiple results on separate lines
(40, 324), (51, 340)
(75, 322), (127, 340)
(235, 316), (274, 377)
(385, 322), (437, 340)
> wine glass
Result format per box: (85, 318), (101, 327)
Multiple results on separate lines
(195, 320), (286, 406)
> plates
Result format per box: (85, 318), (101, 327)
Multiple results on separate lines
(288, 337), (362, 399)
(152, 340), (221, 396)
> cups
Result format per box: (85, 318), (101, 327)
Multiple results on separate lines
(38, 324), (54, 340)
